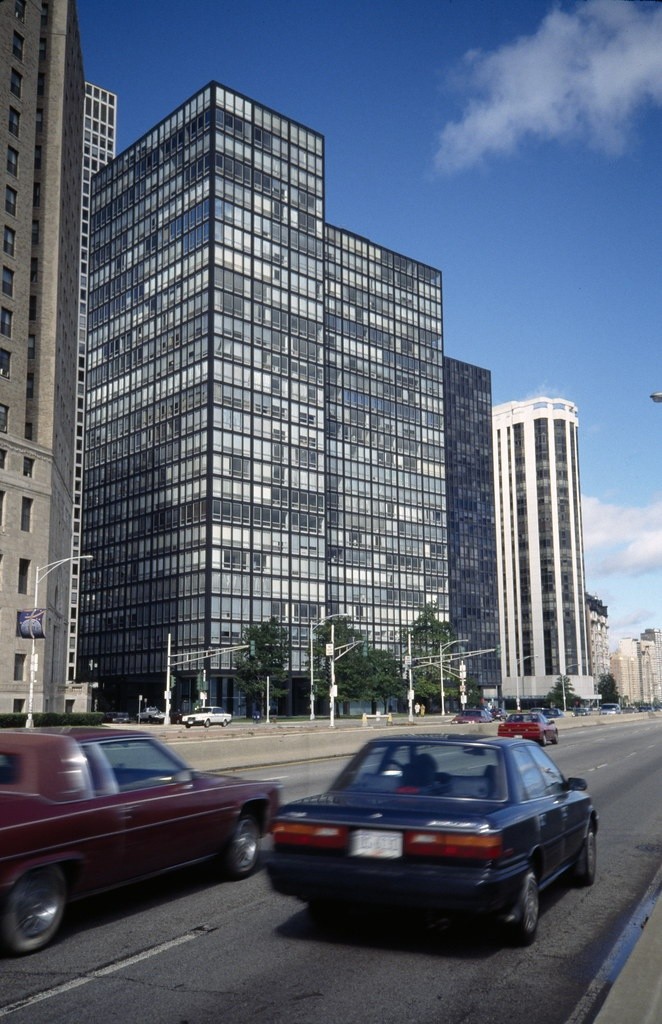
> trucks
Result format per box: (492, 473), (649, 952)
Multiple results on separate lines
(598, 703), (621, 715)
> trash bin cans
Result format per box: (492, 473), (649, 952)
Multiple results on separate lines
(251, 711), (261, 722)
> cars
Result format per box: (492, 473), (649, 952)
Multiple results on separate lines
(543, 708), (566, 719)
(450, 708), (495, 725)
(261, 731), (600, 952)
(181, 706), (232, 728)
(530, 708), (546, 713)
(571, 708), (592, 717)
(0, 727), (282, 956)
(497, 711), (559, 747)
(104, 711), (131, 723)
(489, 708), (508, 721)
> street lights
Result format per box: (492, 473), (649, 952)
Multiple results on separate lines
(23, 553), (95, 730)
(439, 638), (470, 715)
(138, 694), (143, 724)
(514, 653), (540, 712)
(309, 613), (351, 721)
(561, 661), (580, 711)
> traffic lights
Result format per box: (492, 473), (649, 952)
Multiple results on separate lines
(250, 640), (256, 656)
(170, 675), (176, 688)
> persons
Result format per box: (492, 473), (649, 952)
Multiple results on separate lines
(420, 704), (425, 716)
(415, 703), (421, 717)
(410, 752), (439, 786)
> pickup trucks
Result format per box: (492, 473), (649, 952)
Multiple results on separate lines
(137, 707), (166, 724)
